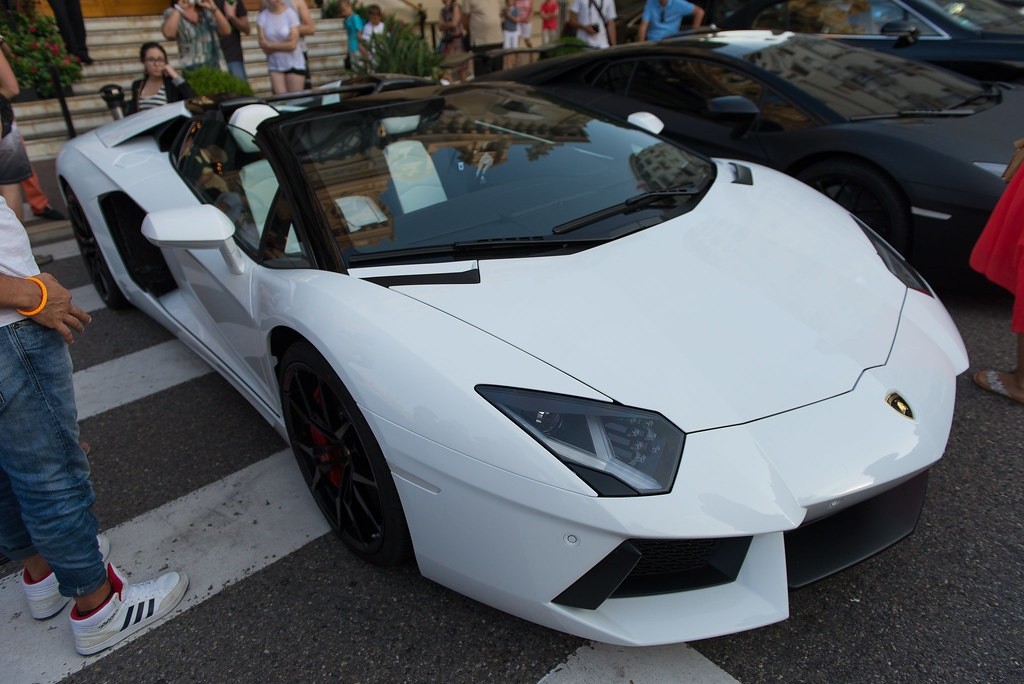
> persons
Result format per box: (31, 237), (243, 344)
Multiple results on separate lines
(504, 0), (534, 70)
(638, 0), (705, 40)
(336, 0), (391, 73)
(541, 0), (558, 42)
(456, 0), (504, 76)
(47, 0), (94, 62)
(439, 0), (465, 55)
(972, 161), (1024, 406)
(161, 0), (231, 74)
(0, 36), (30, 223)
(415, 3), (428, 39)
(569, 0), (619, 49)
(253, 0), (314, 96)
(214, 0), (250, 83)
(20, 138), (69, 221)
(1, 193), (191, 652)
(124, 43), (197, 115)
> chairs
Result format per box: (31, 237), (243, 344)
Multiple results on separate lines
(230, 103), (378, 264)
(377, 82), (461, 208)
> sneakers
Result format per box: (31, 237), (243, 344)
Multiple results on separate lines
(70, 562), (189, 656)
(22, 534), (110, 621)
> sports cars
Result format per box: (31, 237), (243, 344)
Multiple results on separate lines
(463, 27), (1024, 264)
(54, 72), (975, 648)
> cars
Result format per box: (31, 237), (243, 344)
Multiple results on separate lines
(558, 0), (1024, 87)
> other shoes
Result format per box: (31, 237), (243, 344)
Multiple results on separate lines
(33, 206), (64, 220)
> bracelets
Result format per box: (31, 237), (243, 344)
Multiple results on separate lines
(15, 277), (48, 317)
(211, 9), (216, 13)
(173, 4), (183, 13)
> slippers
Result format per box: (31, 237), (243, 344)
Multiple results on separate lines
(973, 370), (1024, 403)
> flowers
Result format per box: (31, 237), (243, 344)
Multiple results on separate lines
(4, 18), (78, 90)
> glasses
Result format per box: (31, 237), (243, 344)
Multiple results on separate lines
(146, 56), (165, 62)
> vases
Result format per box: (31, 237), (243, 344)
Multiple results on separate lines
(11, 86), (39, 102)
(41, 79), (73, 98)
